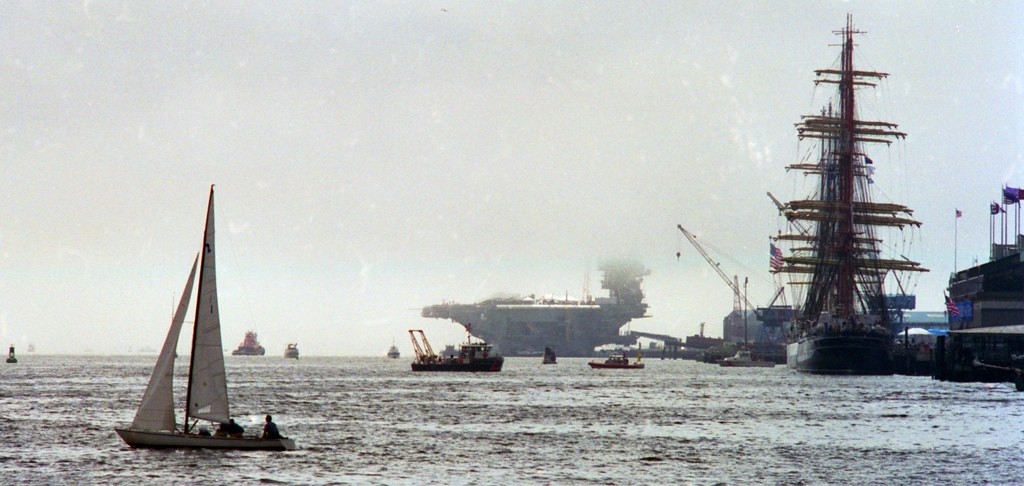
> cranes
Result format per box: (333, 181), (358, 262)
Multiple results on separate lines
(767, 191), (819, 250)
(677, 224), (803, 346)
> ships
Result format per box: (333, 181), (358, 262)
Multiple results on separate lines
(422, 256), (723, 357)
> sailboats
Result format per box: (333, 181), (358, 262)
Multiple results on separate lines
(770, 14), (931, 376)
(114, 184), (296, 450)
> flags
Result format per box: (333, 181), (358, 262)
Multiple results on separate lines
(1002, 186), (1024, 206)
(956, 210), (961, 217)
(768, 245), (783, 273)
(991, 202), (1007, 214)
(944, 295), (959, 314)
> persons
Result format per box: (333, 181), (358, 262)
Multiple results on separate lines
(214, 419), (244, 438)
(262, 415), (278, 439)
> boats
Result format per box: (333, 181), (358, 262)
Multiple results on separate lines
(409, 324), (504, 372)
(388, 337), (400, 358)
(588, 352), (644, 368)
(724, 350), (776, 366)
(231, 330), (265, 355)
(6, 344), (17, 363)
(285, 343), (299, 358)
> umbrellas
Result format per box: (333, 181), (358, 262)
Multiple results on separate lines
(898, 328), (933, 335)
